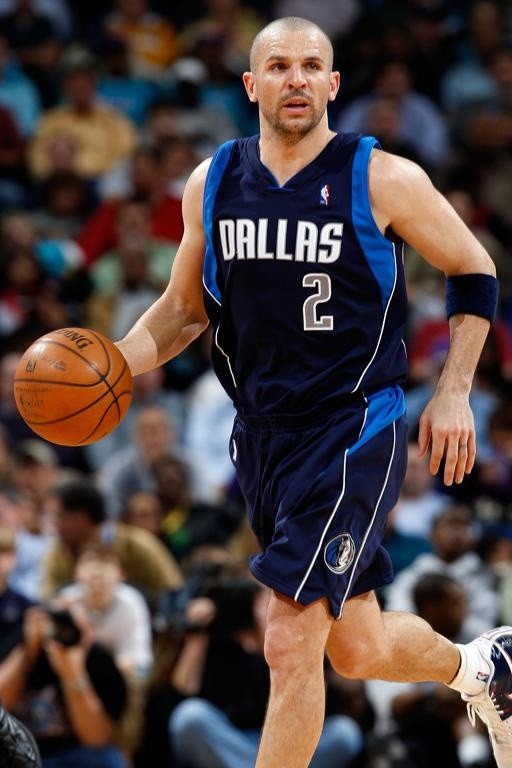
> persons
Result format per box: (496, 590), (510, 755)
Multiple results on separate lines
(106, 15), (512, 767)
(0, 1), (512, 768)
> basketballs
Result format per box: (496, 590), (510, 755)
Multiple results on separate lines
(14, 327), (133, 447)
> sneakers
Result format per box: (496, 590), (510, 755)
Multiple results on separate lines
(462, 626), (512, 768)
